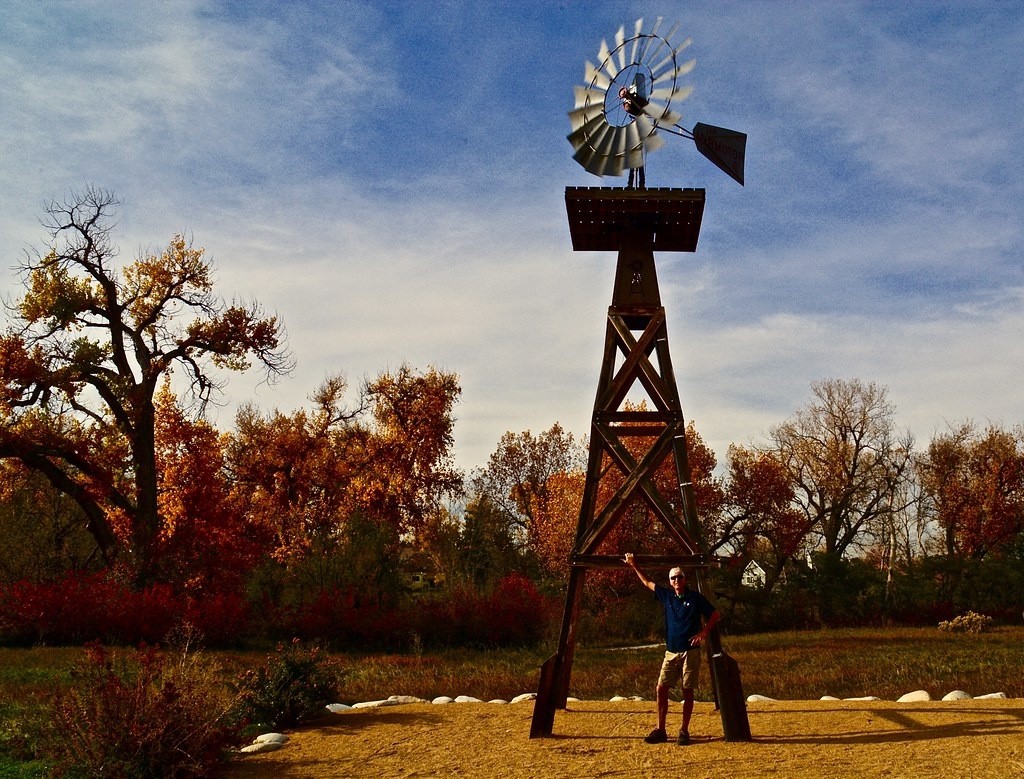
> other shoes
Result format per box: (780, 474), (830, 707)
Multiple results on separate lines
(644, 728), (667, 743)
(678, 730), (689, 745)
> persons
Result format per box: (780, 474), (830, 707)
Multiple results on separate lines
(621, 552), (719, 746)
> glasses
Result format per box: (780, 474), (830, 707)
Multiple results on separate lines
(669, 575), (684, 581)
